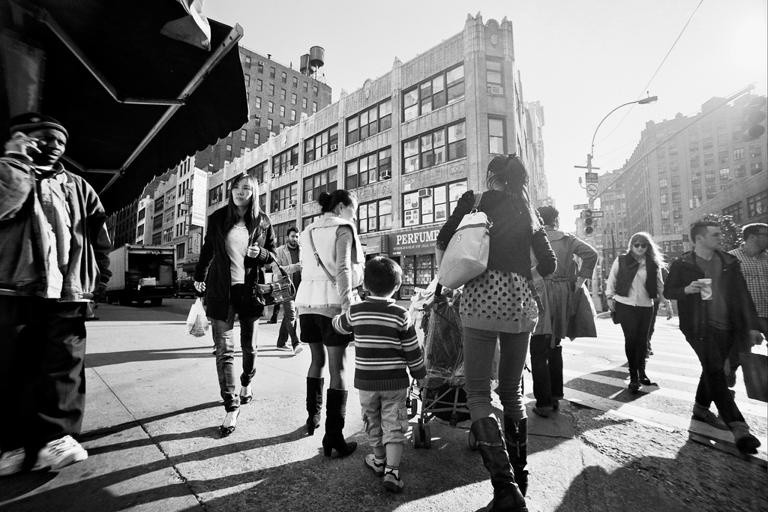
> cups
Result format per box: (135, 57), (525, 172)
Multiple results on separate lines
(697, 278), (713, 301)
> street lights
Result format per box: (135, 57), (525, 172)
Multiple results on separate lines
(587, 95), (658, 312)
(591, 95), (658, 157)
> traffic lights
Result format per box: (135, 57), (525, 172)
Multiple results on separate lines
(585, 210), (594, 234)
(582, 210), (593, 235)
(743, 94), (767, 143)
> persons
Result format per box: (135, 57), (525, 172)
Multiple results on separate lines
(529, 206), (600, 418)
(726, 222), (768, 399)
(662, 219), (764, 456)
(435, 153), (558, 512)
(330, 254), (427, 493)
(604, 230), (674, 396)
(270, 226), (304, 356)
(194, 173), (279, 438)
(294, 189), (366, 459)
(0, 110), (112, 477)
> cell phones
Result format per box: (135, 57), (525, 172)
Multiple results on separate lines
(11, 130), (38, 150)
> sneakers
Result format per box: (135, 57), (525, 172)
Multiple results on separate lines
(86, 317), (99, 320)
(639, 375), (650, 385)
(534, 405), (552, 416)
(552, 398), (559, 410)
(383, 472), (403, 491)
(1, 449), (26, 474)
(630, 383), (639, 394)
(727, 422), (762, 454)
(364, 454), (384, 477)
(267, 320), (276, 323)
(691, 403), (729, 430)
(295, 345), (303, 354)
(32, 435), (88, 471)
(278, 345), (292, 351)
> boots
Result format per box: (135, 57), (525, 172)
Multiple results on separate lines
(504, 415), (528, 496)
(470, 417), (527, 512)
(306, 377), (324, 435)
(323, 389), (357, 457)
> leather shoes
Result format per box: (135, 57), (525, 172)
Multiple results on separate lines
(220, 410), (240, 436)
(240, 389), (253, 404)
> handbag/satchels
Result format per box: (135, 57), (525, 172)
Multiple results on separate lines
(611, 302), (620, 324)
(437, 191), (494, 290)
(739, 340), (768, 403)
(252, 251), (296, 306)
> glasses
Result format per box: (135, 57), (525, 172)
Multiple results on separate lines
(631, 243), (647, 248)
(751, 232), (768, 235)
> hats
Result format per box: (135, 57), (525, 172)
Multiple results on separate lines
(9, 112), (70, 138)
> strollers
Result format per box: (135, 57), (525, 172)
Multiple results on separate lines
(400, 273), (501, 451)
